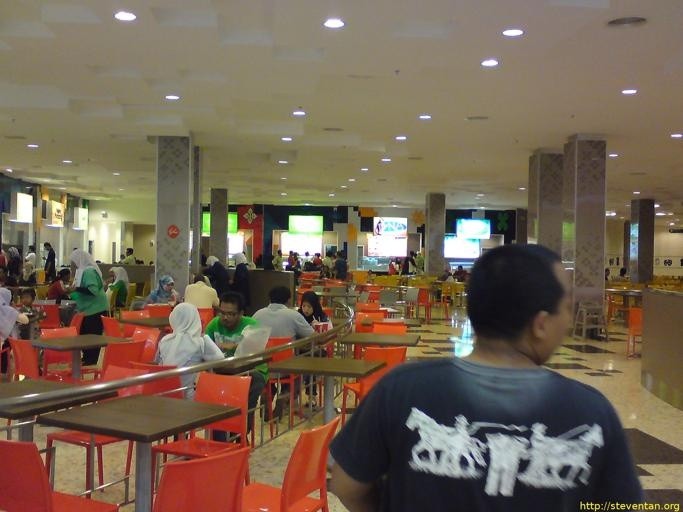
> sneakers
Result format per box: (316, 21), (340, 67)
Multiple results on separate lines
(259, 395), (284, 422)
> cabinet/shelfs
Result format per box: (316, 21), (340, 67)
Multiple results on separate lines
(281, 256), (314, 271)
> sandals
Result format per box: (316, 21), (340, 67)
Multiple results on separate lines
(307, 394), (317, 406)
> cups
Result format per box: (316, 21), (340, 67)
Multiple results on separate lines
(310, 321), (328, 333)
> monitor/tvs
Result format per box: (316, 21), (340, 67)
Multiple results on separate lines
(442, 233), (480, 260)
(455, 218), (491, 240)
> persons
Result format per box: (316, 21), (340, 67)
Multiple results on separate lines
(612, 267), (628, 280)
(604, 268), (610, 280)
(327, 242), (646, 512)
(0, 234), (468, 445)
